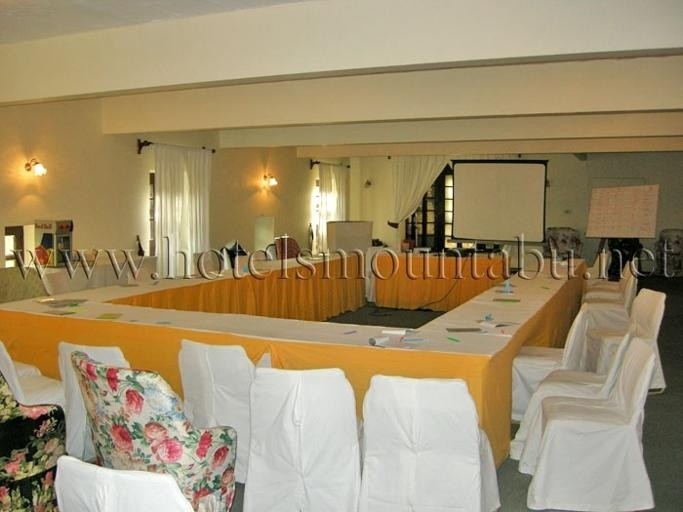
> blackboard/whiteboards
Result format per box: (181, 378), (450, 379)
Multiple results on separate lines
(327, 221), (373, 252)
(584, 184), (659, 238)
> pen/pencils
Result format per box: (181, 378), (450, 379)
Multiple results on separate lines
(345, 330), (357, 335)
(402, 338), (423, 341)
(447, 337), (459, 343)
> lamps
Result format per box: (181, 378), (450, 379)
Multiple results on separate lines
(24, 158), (46, 177)
(262, 174), (278, 186)
